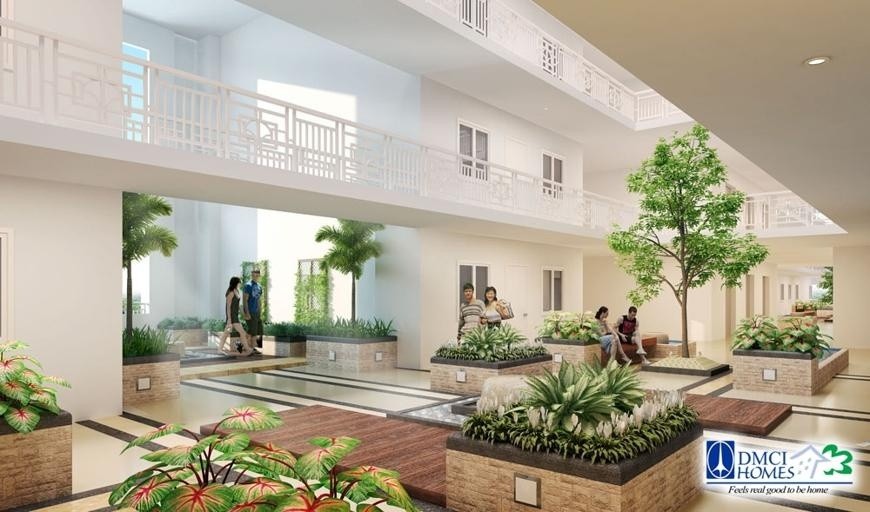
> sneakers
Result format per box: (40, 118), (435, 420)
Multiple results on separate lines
(637, 349), (647, 355)
(642, 360), (651, 364)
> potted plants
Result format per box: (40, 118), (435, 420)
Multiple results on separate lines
(0, 119), (851, 512)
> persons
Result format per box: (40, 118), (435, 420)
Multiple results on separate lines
(591, 306), (631, 367)
(218, 277), (252, 355)
(243, 270), (262, 349)
(459, 282), (485, 340)
(615, 306), (652, 364)
(481, 287), (503, 329)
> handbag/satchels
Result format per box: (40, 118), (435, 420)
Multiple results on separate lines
(496, 299), (515, 320)
